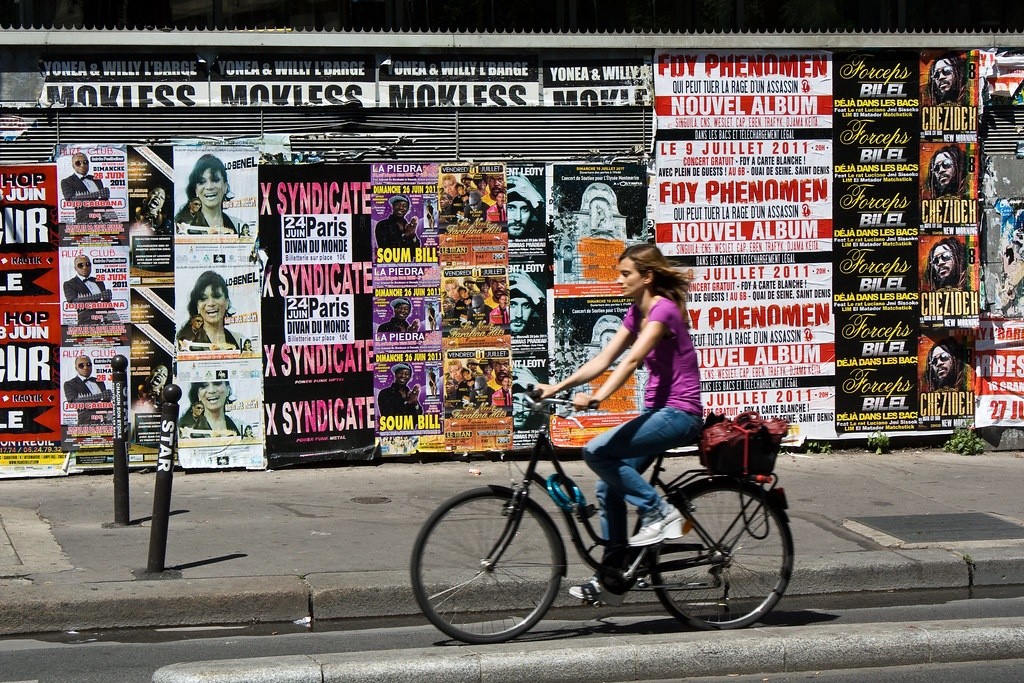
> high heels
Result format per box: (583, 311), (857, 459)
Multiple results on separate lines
(628, 507), (685, 547)
(568, 571), (630, 607)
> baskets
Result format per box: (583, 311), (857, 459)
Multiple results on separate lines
(699, 432), (783, 476)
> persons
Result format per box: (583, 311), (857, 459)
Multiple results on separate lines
(63, 256), (120, 325)
(534, 245), (703, 606)
(64, 355), (114, 424)
(175, 155), (256, 237)
(132, 185), (170, 260)
(176, 381), (256, 440)
(926, 337), (966, 394)
(376, 171), (545, 429)
(61, 153), (119, 224)
(922, 54), (970, 108)
(131, 364), (168, 435)
(925, 238), (971, 301)
(178, 270), (256, 353)
(923, 143), (969, 199)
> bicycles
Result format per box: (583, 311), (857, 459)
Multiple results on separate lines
(410, 396), (797, 645)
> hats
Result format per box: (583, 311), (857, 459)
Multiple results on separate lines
(461, 173), (473, 180)
(390, 362), (413, 377)
(466, 358), (479, 367)
(463, 277), (476, 284)
(390, 297), (411, 308)
(389, 194), (409, 204)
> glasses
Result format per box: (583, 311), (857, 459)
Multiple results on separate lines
(75, 160), (89, 166)
(474, 385), (485, 391)
(77, 262), (91, 269)
(197, 407), (205, 411)
(933, 64), (952, 80)
(932, 157), (953, 173)
(196, 319), (203, 324)
(77, 363), (93, 369)
(470, 302), (482, 309)
(931, 250), (953, 267)
(468, 201), (478, 206)
(929, 351), (950, 367)
(194, 202), (201, 207)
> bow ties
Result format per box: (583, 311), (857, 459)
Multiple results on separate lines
(81, 175), (93, 180)
(83, 378), (94, 383)
(83, 277), (94, 283)
